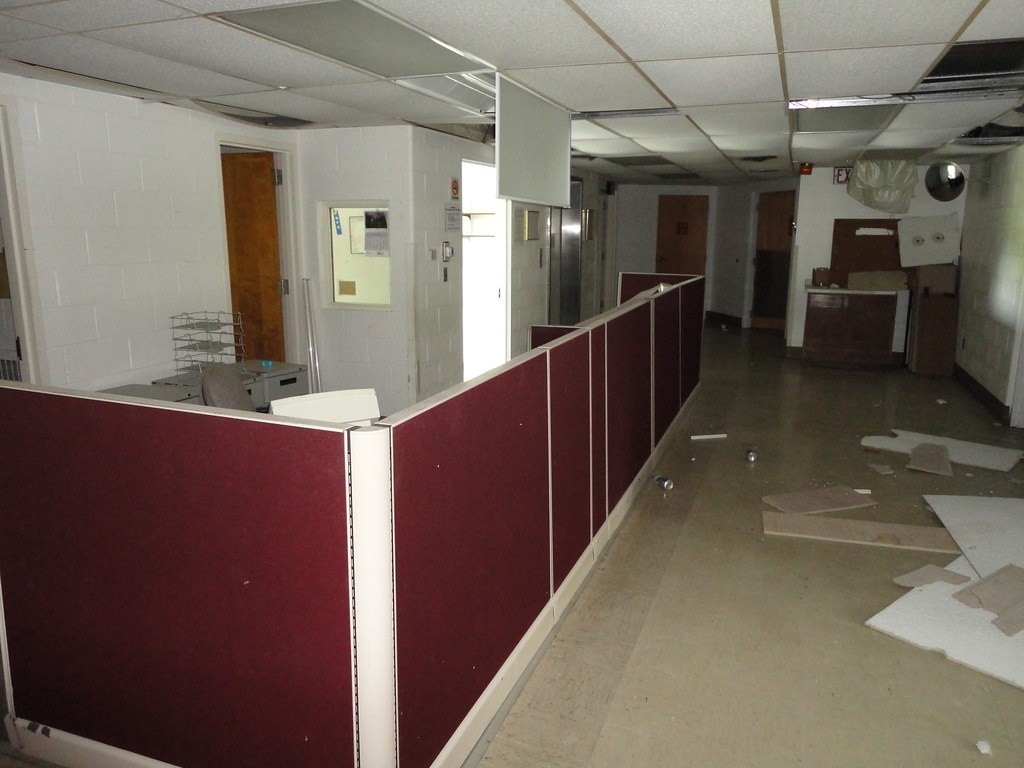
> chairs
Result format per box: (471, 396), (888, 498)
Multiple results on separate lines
(202, 363), (256, 412)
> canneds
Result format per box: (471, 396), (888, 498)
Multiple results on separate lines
(744, 449), (757, 461)
(654, 475), (673, 489)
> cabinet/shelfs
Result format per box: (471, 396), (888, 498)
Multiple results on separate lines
(905, 265), (960, 377)
(170, 310), (246, 386)
(101, 359), (309, 406)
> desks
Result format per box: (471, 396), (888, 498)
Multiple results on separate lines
(802, 284), (897, 370)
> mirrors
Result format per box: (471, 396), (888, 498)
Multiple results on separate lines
(925, 160), (966, 202)
